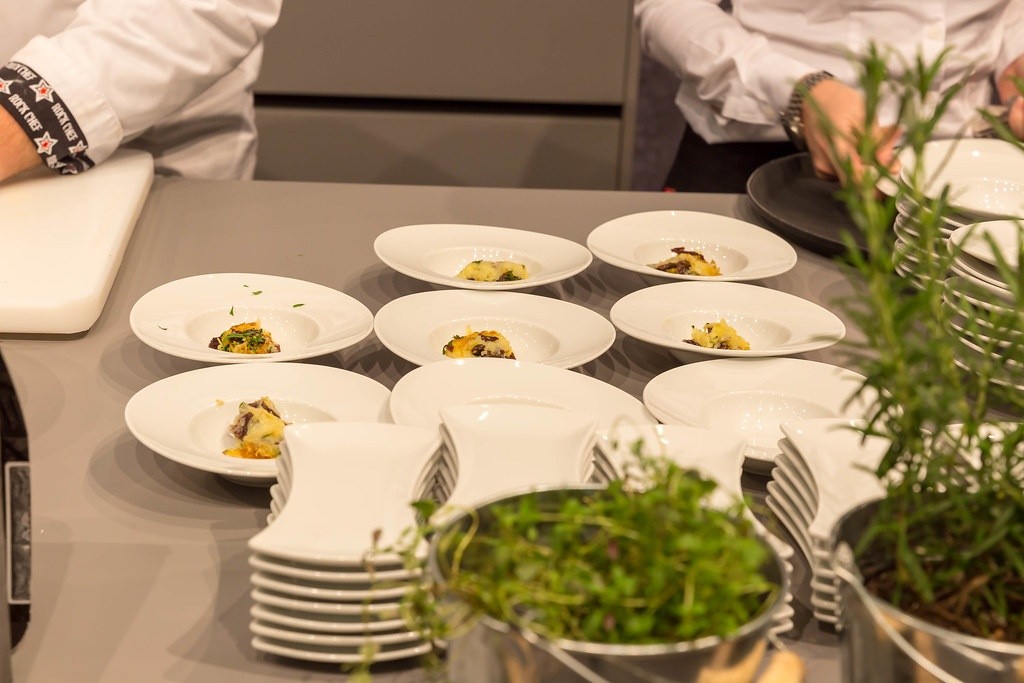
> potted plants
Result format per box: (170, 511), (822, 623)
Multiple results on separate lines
(356, 38), (1024, 683)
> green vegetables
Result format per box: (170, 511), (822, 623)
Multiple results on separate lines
(338, 432), (783, 683)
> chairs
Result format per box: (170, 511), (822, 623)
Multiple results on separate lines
(0, 181), (881, 683)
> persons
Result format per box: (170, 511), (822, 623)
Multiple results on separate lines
(633, 0), (1024, 200)
(0, 0), (283, 184)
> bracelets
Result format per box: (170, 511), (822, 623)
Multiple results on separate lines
(779, 70), (834, 150)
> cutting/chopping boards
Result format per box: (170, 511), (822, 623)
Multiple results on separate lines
(0, 146), (153, 333)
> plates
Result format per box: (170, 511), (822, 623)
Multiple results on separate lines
(123, 135), (1024, 664)
